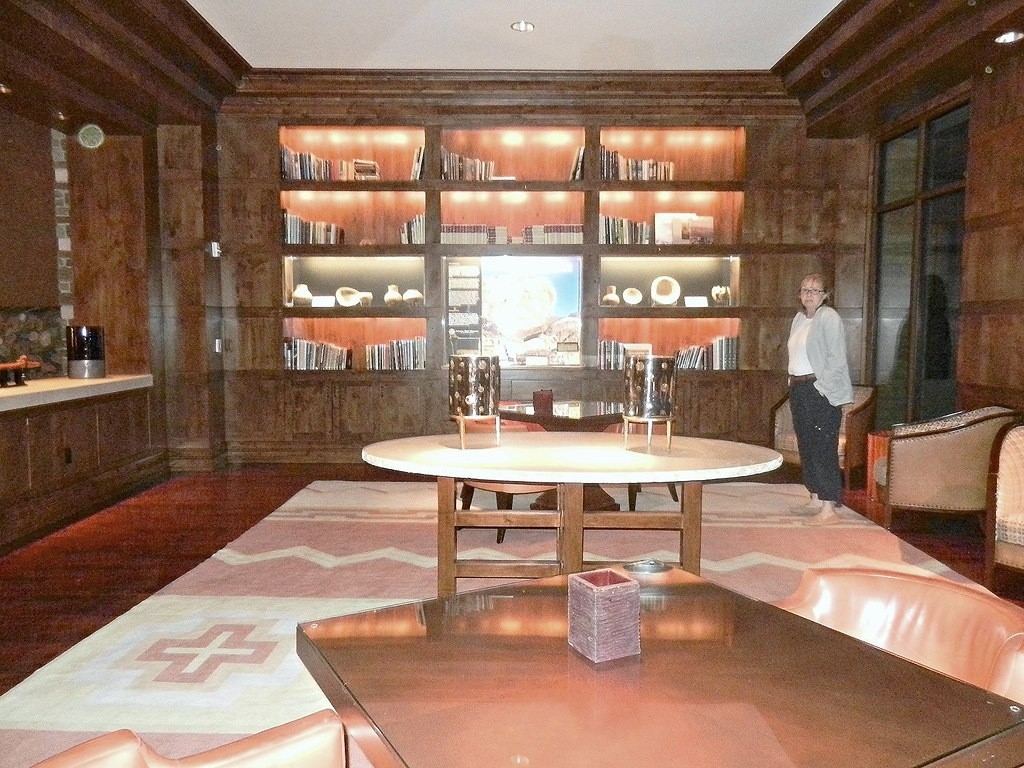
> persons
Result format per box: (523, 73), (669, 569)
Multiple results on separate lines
(284, 336), (353, 369)
(787, 273), (853, 527)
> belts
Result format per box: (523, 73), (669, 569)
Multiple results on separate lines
(790, 373), (816, 381)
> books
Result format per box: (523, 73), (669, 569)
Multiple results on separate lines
(280, 145), (380, 180)
(599, 211), (713, 245)
(674, 336), (738, 371)
(283, 207), (345, 245)
(400, 212), (425, 244)
(600, 144), (674, 181)
(441, 145), (516, 180)
(568, 148), (584, 181)
(365, 336), (427, 369)
(411, 146), (425, 179)
(440, 224), (584, 244)
(596, 339), (625, 371)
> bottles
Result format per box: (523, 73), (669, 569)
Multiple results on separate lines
(384, 285), (402, 307)
(402, 289), (424, 307)
(682, 223), (689, 244)
(603, 286), (620, 305)
(291, 284), (312, 306)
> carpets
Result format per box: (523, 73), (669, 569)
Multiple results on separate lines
(0, 481), (1024, 768)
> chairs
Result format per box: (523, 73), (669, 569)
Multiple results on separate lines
(23, 707), (345, 768)
(446, 409), (557, 543)
(770, 384), (877, 494)
(882, 406), (1024, 593)
(600, 423), (679, 510)
(761, 567), (1024, 707)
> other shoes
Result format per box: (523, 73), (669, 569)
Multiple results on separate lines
(803, 511), (840, 526)
(789, 503), (822, 514)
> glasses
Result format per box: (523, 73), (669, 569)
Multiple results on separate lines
(800, 288), (825, 294)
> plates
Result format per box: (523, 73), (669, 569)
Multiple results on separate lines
(650, 276), (681, 304)
(623, 288), (642, 304)
(336, 286), (360, 306)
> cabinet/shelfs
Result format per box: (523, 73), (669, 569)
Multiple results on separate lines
(214, 67), (807, 463)
(0, 387), (171, 543)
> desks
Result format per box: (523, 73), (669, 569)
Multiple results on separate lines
(296, 559), (1024, 768)
(451, 398), (628, 513)
(360, 432), (784, 598)
